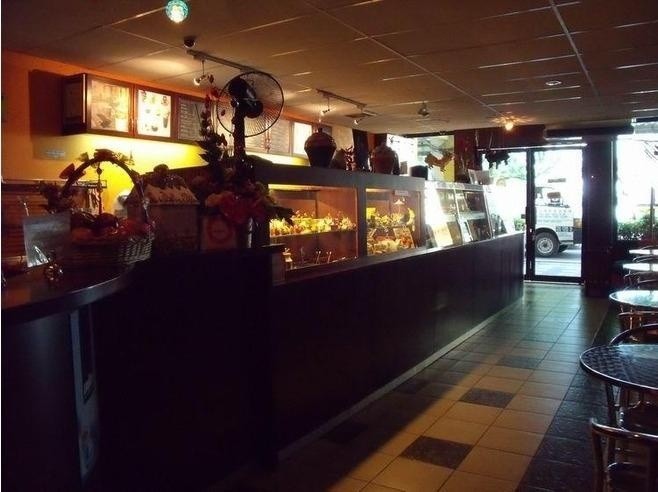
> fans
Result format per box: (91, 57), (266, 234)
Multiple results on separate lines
(216, 71), (284, 164)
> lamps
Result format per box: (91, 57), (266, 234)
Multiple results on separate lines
(418, 106), (430, 119)
(316, 89), (367, 126)
(193, 60), (208, 86)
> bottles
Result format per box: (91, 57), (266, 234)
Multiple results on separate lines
(163, 117), (168, 128)
(303, 127), (338, 169)
(372, 140), (396, 177)
(283, 252), (293, 270)
(473, 223), (487, 241)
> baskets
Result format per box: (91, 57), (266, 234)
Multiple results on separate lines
(52, 157), (153, 265)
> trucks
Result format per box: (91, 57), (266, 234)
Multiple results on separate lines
(534, 185), (574, 256)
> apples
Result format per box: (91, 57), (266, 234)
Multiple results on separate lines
(73, 212), (138, 243)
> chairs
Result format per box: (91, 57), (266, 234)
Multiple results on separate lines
(580, 246), (658, 492)
